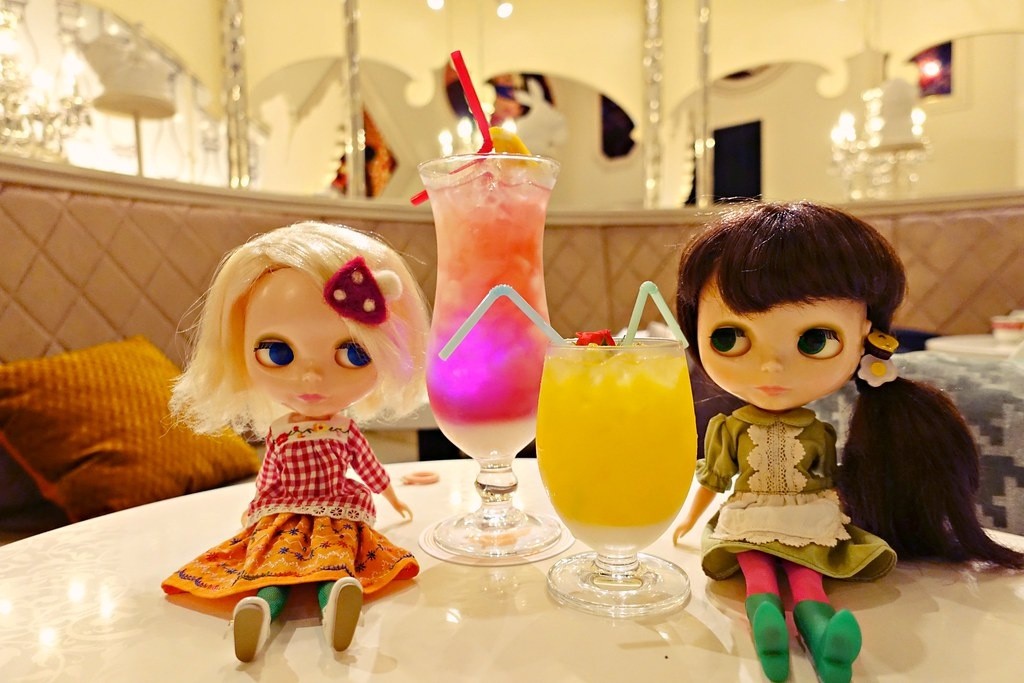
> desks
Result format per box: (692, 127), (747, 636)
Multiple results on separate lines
(0, 453), (1024, 683)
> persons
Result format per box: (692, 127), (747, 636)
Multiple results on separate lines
(664, 201), (1024, 683)
(158, 221), (435, 662)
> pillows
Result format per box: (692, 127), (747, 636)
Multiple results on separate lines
(0, 331), (261, 525)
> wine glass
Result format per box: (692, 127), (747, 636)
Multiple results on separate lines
(535, 338), (698, 616)
(417, 153), (576, 567)
